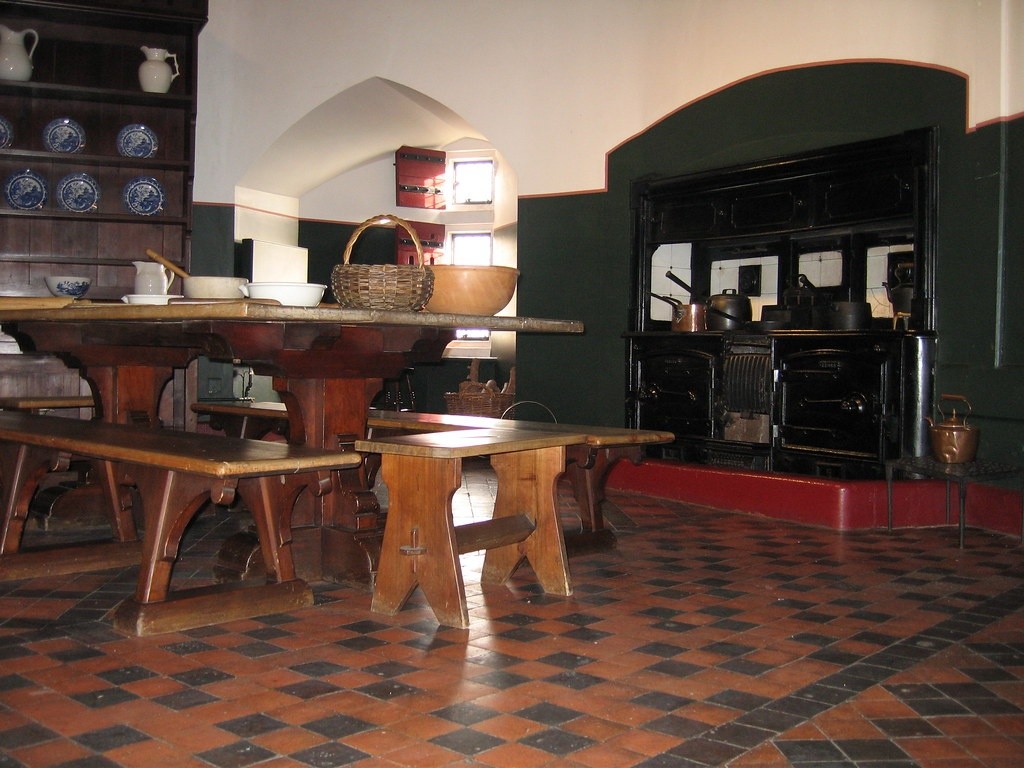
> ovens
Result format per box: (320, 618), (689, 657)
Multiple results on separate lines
(771, 336), (900, 480)
(623, 335), (715, 438)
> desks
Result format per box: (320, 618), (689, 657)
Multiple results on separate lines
(0, 297), (589, 589)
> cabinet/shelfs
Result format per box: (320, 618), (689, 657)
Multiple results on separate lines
(0, 0), (211, 302)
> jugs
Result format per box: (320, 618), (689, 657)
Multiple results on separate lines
(0, 24), (39, 81)
(137, 45), (181, 94)
(131, 261), (175, 295)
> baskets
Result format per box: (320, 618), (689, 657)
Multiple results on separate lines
(330, 214), (434, 311)
(443, 383), (515, 419)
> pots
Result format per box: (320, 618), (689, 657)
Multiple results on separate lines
(665, 271), (752, 332)
(646, 291), (707, 332)
(790, 274), (873, 331)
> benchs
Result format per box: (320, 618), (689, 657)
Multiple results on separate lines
(0, 395), (95, 411)
(0, 409), (366, 642)
(189, 399), (677, 558)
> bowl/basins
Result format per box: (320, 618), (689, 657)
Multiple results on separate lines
(182, 277), (249, 299)
(238, 282), (328, 308)
(44, 276), (93, 300)
(424, 264), (521, 317)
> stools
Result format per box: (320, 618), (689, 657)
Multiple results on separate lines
(880, 455), (1024, 549)
(349, 426), (584, 632)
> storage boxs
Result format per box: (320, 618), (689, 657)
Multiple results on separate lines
(724, 410), (770, 445)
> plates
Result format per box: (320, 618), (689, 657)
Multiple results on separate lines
(115, 123), (159, 159)
(55, 170), (102, 214)
(122, 175), (168, 217)
(0, 115), (15, 149)
(42, 118), (87, 154)
(126, 294), (185, 305)
(3, 169), (49, 211)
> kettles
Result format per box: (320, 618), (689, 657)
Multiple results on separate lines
(882, 263), (914, 314)
(924, 394), (981, 465)
